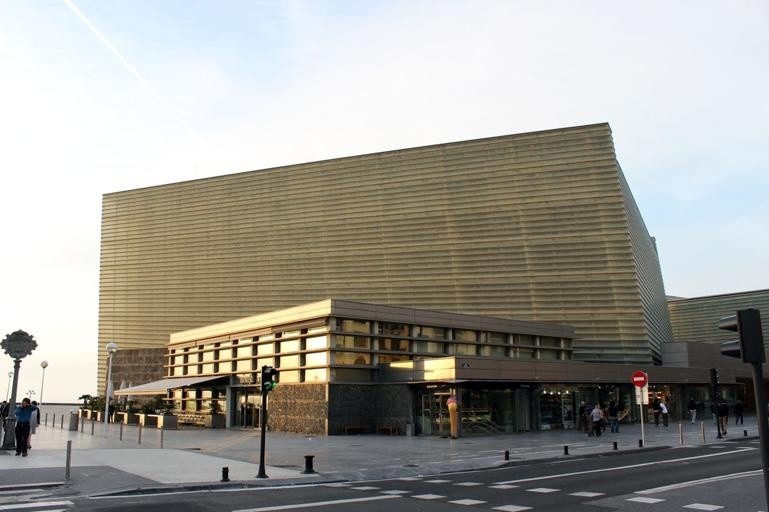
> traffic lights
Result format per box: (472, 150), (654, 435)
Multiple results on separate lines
(716, 312), (741, 358)
(264, 368), (278, 390)
(716, 368), (719, 384)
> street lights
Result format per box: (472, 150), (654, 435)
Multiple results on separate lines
(25, 389), (35, 400)
(5, 371), (12, 399)
(104, 342), (117, 437)
(39, 361), (48, 420)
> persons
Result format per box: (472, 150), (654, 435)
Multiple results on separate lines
(107, 400), (116, 425)
(573, 394), (746, 437)
(0, 397), (40, 460)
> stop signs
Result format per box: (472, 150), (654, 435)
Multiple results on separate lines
(632, 370), (647, 388)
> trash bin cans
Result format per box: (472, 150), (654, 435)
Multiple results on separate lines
(406, 423), (416, 436)
(70, 414), (78, 431)
